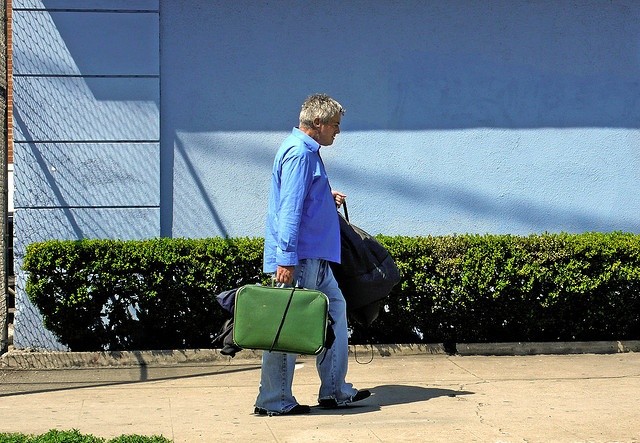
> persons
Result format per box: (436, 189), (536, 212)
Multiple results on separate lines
(254, 93), (371, 415)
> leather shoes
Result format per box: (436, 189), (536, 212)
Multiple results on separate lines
(318, 388), (370, 408)
(254, 405), (310, 415)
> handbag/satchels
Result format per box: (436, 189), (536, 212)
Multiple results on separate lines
(329, 199), (400, 310)
(233, 276), (330, 354)
